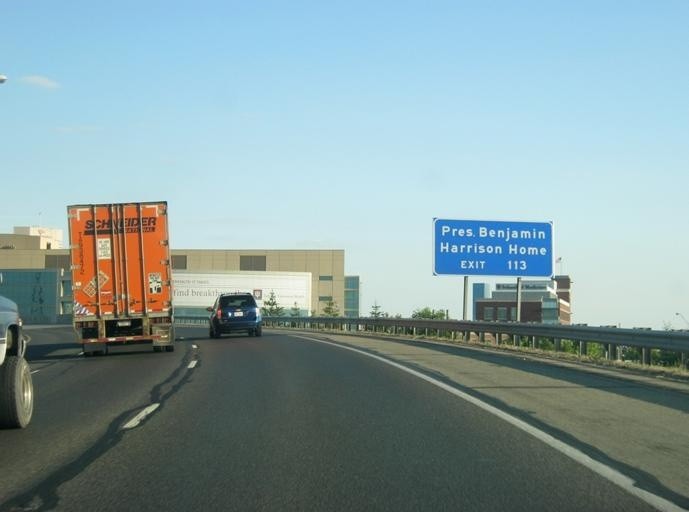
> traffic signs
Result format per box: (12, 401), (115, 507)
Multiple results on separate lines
(432, 221), (554, 278)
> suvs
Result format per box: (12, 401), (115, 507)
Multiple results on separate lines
(0, 270), (34, 436)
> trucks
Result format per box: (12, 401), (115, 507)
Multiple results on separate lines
(66, 201), (175, 356)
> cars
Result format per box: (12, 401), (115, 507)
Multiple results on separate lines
(206, 288), (265, 340)
